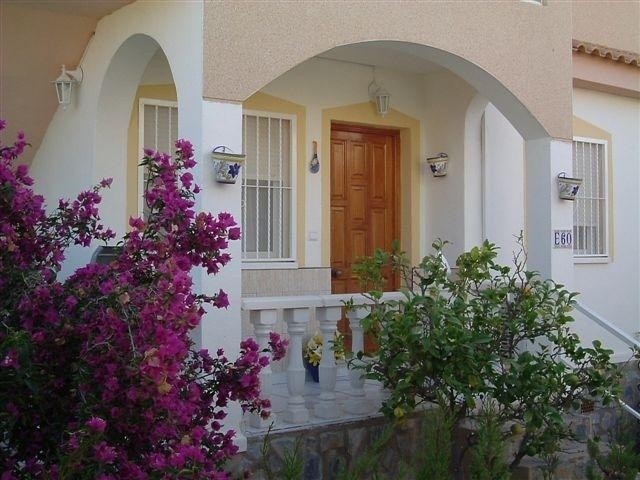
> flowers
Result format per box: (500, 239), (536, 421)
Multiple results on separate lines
(304, 329), (347, 366)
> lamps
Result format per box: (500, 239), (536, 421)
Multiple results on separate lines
(368, 83), (395, 117)
(50, 65), (84, 110)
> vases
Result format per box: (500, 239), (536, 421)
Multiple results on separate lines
(308, 366), (325, 383)
